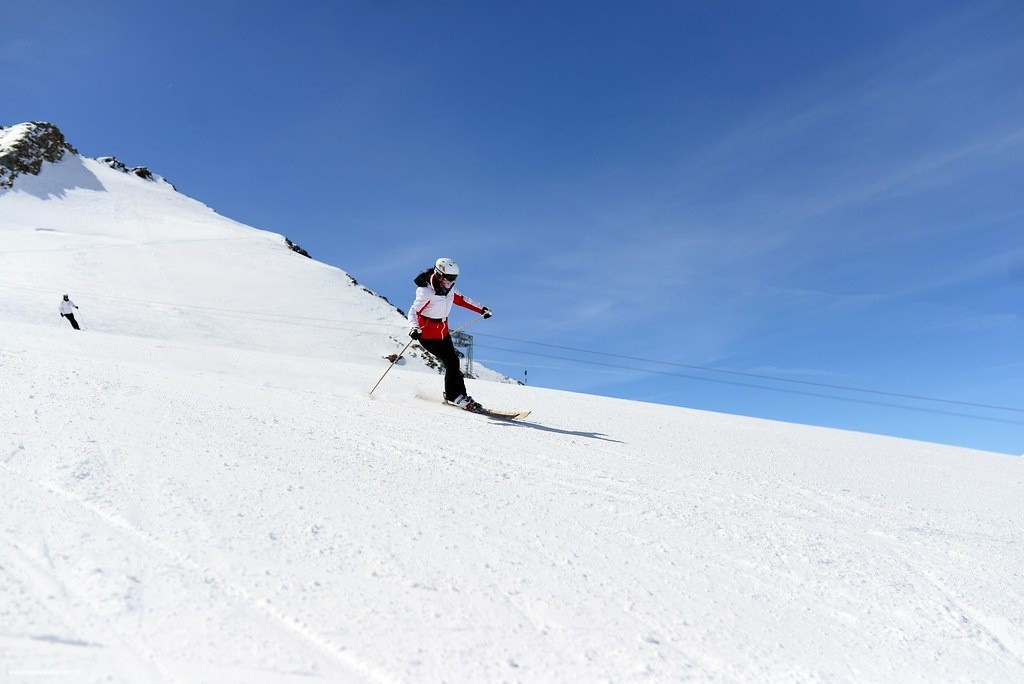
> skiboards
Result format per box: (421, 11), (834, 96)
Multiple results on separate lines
(413, 390), (531, 421)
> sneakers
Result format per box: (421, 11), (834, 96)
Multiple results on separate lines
(442, 392), (488, 414)
(464, 396), (482, 408)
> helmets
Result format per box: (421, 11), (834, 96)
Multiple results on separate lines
(430, 257), (459, 295)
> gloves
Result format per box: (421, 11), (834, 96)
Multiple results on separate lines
(409, 327), (422, 340)
(483, 306), (492, 319)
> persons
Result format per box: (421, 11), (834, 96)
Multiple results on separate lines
(407, 258), (494, 411)
(58, 293), (81, 330)
